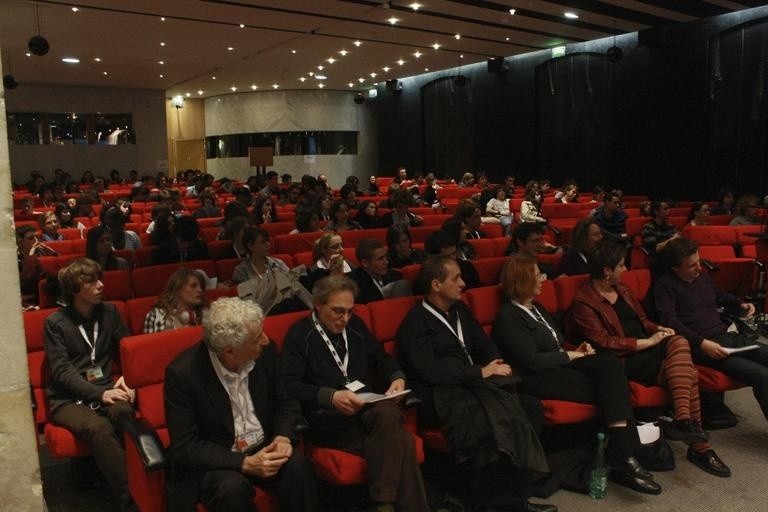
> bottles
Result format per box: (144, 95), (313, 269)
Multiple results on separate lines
(587, 432), (609, 498)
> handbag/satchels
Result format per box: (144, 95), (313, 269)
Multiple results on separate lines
(638, 438), (674, 471)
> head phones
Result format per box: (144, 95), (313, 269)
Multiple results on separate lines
(177, 296), (197, 324)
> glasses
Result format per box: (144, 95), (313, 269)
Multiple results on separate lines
(332, 307), (356, 319)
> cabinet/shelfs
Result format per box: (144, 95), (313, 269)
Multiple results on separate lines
(168, 133), (206, 181)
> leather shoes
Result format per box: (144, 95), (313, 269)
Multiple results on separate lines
(665, 418), (708, 443)
(610, 455), (662, 494)
(686, 447), (731, 477)
(526, 502), (557, 512)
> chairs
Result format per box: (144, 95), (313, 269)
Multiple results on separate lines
(10, 176), (766, 509)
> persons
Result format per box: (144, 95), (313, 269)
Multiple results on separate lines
(16, 167), (222, 335)
(653, 238), (767, 421)
(392, 257), (558, 512)
(218, 168), (486, 316)
(566, 239), (730, 477)
(163, 296), (322, 512)
(489, 255), (662, 495)
(44, 258), (140, 512)
(277, 275), (430, 512)
(460, 171), (767, 281)
